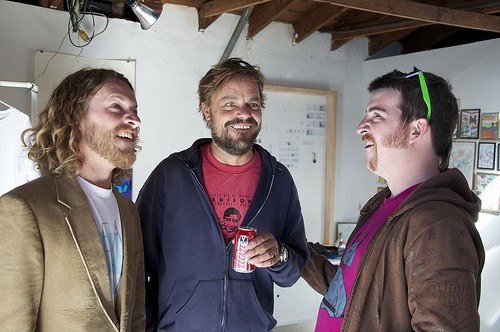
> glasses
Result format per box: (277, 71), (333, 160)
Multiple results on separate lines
(402, 69), (432, 124)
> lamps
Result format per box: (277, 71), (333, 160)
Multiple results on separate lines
(124, 0), (163, 30)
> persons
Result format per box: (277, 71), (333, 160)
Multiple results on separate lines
(135, 58), (310, 332)
(0, 68), (147, 332)
(301, 67), (486, 332)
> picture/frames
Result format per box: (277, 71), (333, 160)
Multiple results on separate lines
(447, 108), (500, 215)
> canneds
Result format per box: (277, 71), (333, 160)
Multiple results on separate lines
(232, 227), (256, 273)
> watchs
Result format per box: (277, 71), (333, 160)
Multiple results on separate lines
(273, 241), (288, 268)
